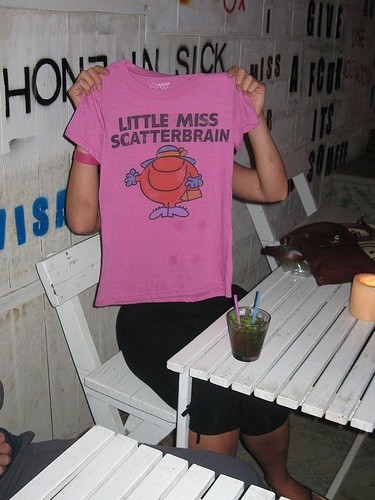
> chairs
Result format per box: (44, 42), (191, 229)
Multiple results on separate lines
(245, 147), (318, 272)
(35, 233), (177, 446)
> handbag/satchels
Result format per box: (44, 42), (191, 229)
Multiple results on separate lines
(282, 222), (375, 287)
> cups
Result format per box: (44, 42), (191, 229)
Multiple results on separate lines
(228, 306), (271, 362)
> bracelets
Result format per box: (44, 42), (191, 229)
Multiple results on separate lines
(73, 149), (99, 165)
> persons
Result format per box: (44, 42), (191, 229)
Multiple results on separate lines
(66, 65), (322, 500)
(0, 379), (263, 500)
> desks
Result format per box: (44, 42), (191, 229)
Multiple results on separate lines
(166, 264), (375, 500)
(8, 424), (292, 500)
